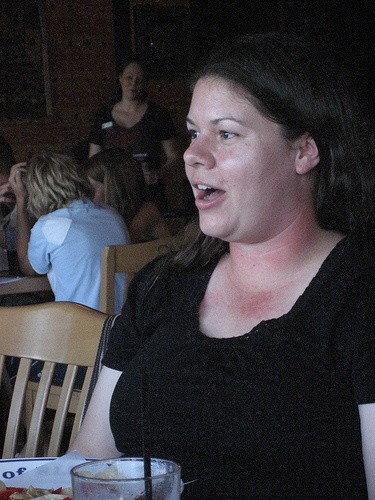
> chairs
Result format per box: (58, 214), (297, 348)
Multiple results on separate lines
(0, 233), (200, 459)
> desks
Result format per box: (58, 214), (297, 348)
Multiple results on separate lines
(0, 268), (51, 295)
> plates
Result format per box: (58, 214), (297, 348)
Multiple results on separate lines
(0, 457), (184, 500)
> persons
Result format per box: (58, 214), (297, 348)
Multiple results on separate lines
(79, 150), (170, 244)
(88, 56), (181, 215)
(11, 148), (132, 392)
(66, 31), (375, 500)
(1, 139), (36, 281)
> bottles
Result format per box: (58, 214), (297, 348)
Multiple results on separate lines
(0, 229), (9, 277)
(142, 152), (153, 175)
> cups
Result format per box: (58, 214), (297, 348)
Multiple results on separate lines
(70, 457), (182, 500)
(7, 250), (24, 277)
